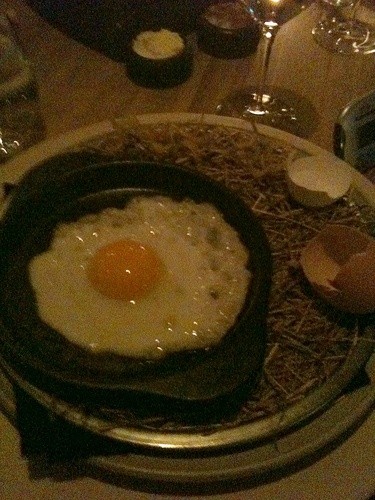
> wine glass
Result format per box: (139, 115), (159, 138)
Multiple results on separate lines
(216, 0), (314, 135)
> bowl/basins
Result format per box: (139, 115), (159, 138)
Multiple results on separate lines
(126, 24), (191, 86)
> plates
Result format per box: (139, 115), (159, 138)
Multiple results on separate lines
(332, 88), (375, 189)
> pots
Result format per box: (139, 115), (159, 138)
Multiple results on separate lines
(1, 150), (276, 405)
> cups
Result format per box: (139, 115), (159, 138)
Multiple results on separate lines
(310, 0), (374, 56)
(191, 0), (258, 57)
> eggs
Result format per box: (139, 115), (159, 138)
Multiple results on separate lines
(132, 28), (185, 60)
(28, 193), (250, 359)
